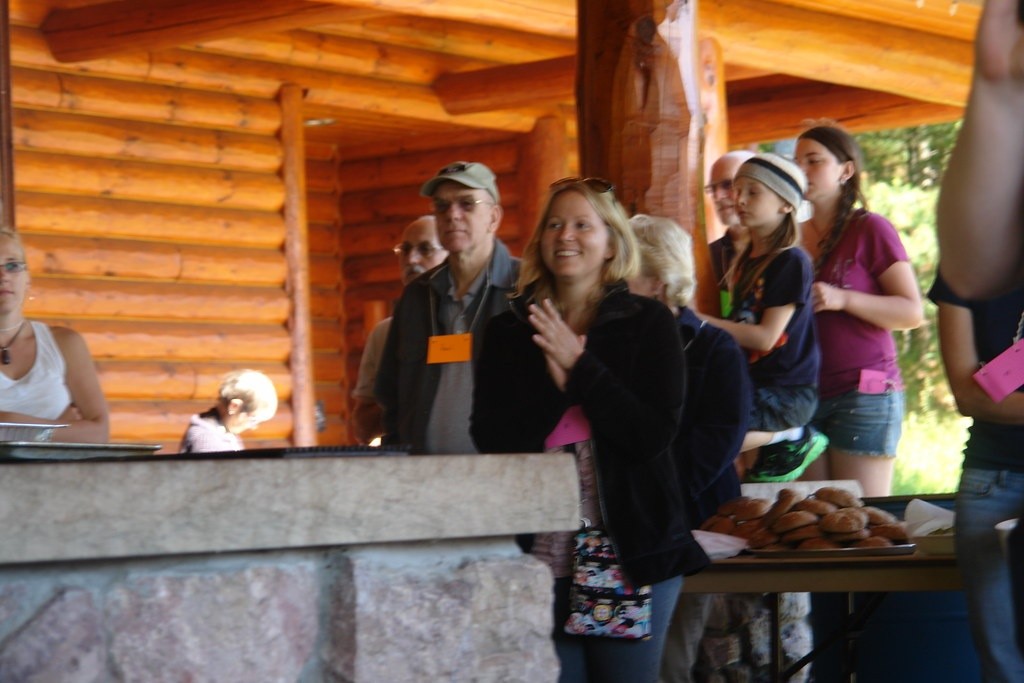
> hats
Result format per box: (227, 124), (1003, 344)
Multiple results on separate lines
(420, 161), (500, 204)
(733, 151), (809, 208)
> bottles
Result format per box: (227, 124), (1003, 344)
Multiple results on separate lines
(737, 302), (754, 325)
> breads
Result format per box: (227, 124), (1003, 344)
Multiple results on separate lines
(701, 487), (907, 549)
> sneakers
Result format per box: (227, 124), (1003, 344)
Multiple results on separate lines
(748, 424), (831, 483)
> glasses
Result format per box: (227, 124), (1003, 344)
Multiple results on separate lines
(393, 242), (445, 257)
(0, 262), (26, 272)
(428, 197), (495, 215)
(549, 176), (616, 207)
(704, 179), (735, 195)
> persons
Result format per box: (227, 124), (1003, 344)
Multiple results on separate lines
(178, 369), (279, 453)
(925, 0), (1024, 683)
(343, 149), (829, 683)
(0, 226), (110, 445)
(794, 125), (927, 497)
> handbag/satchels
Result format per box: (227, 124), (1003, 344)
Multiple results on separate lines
(563, 527), (654, 642)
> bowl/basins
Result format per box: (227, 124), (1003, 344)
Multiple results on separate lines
(912, 534), (957, 556)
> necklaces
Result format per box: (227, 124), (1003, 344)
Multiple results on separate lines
(0, 319), (27, 364)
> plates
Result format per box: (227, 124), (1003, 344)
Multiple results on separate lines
(0, 422), (72, 443)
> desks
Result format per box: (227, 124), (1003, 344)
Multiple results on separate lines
(679, 533), (996, 683)
